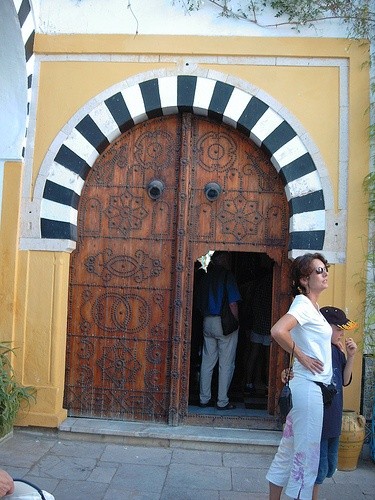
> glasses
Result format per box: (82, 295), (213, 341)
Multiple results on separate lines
(307, 266), (328, 277)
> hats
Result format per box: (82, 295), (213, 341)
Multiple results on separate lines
(318, 306), (358, 330)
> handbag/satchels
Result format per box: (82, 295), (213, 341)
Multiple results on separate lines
(220, 293), (238, 336)
(279, 386), (292, 416)
(314, 380), (338, 408)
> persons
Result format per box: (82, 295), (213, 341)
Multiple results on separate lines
(281, 305), (357, 500)
(189, 251), (272, 412)
(0, 469), (55, 500)
(265, 252), (334, 499)
(243, 257), (270, 391)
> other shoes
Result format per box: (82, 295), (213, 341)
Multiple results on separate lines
(200, 399), (215, 407)
(217, 403), (237, 410)
